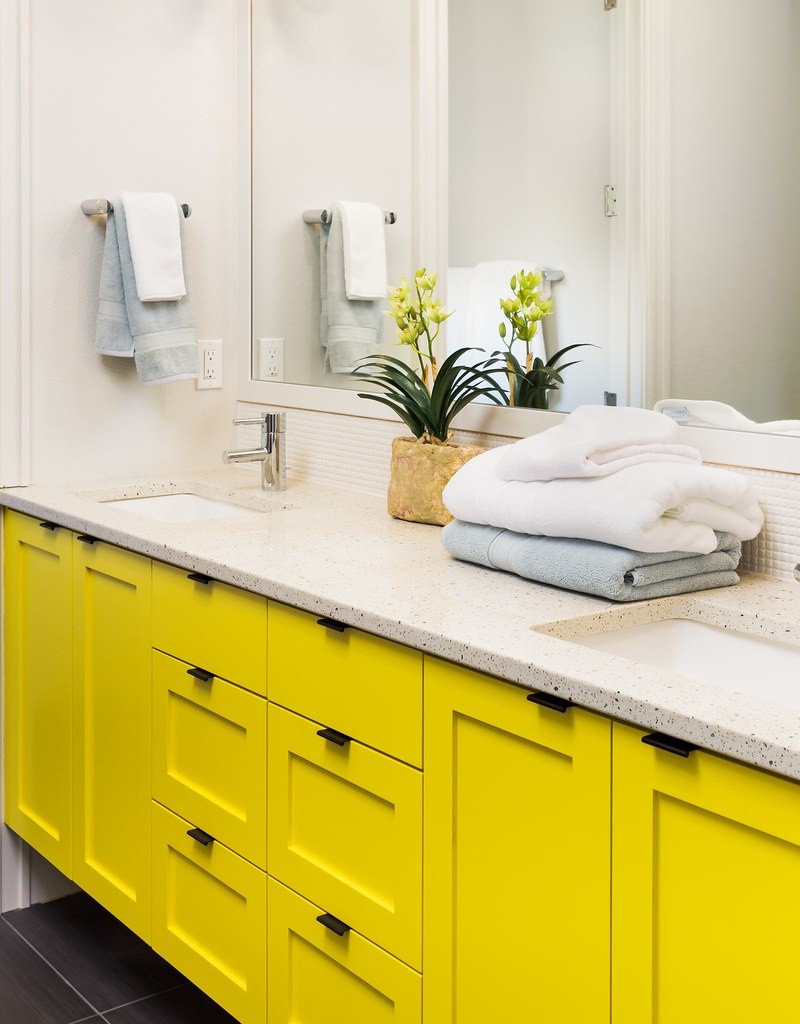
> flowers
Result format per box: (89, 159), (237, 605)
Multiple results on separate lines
(349, 266), (533, 445)
(458, 268), (602, 409)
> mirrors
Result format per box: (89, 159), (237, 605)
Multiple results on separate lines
(249, 1), (800, 439)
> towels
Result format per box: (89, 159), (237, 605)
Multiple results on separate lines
(446, 258), (552, 407)
(328, 200), (388, 302)
(319, 205), (388, 377)
(93, 197), (201, 387)
(440, 443), (765, 555)
(117, 191), (188, 303)
(439, 518), (742, 602)
(494, 404), (704, 483)
(652, 397), (800, 438)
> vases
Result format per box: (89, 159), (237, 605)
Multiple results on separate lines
(387, 436), (485, 527)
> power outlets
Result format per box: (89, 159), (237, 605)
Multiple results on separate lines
(259, 338), (284, 382)
(196, 339), (223, 389)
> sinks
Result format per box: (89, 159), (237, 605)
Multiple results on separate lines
(96, 493), (269, 523)
(559, 617), (800, 711)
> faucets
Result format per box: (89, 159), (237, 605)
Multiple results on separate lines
(222, 411), (287, 493)
(793, 563), (800, 583)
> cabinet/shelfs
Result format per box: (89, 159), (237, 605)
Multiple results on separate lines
(2, 508), (799, 1023)
